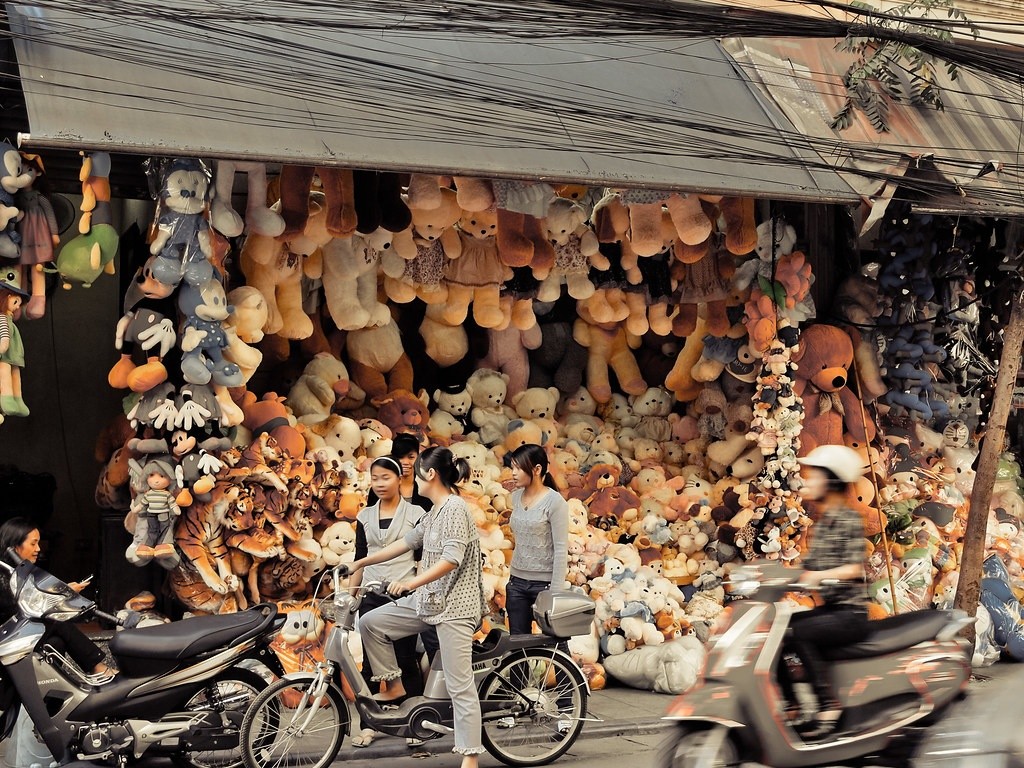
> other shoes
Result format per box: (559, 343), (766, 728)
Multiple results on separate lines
(373, 693), (407, 705)
(800, 709), (841, 738)
(786, 713), (817, 737)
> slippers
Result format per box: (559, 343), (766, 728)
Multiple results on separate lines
(557, 716), (573, 732)
(349, 733), (375, 749)
(407, 738), (427, 747)
(497, 717), (515, 728)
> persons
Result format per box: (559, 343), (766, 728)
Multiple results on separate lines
(775, 444), (869, 745)
(494, 446), (581, 733)
(331, 445), (490, 767)
(347, 455), (427, 747)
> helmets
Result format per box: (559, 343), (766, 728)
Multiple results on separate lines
(792, 443), (863, 482)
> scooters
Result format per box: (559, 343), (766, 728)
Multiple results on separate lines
(652, 542), (975, 768)
(235, 561), (598, 768)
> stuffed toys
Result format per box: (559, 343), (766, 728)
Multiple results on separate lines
(0, 144), (1023, 709)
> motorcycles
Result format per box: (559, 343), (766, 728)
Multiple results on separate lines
(0, 545), (289, 768)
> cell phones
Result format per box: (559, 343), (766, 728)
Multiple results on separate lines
(78, 574), (94, 584)
